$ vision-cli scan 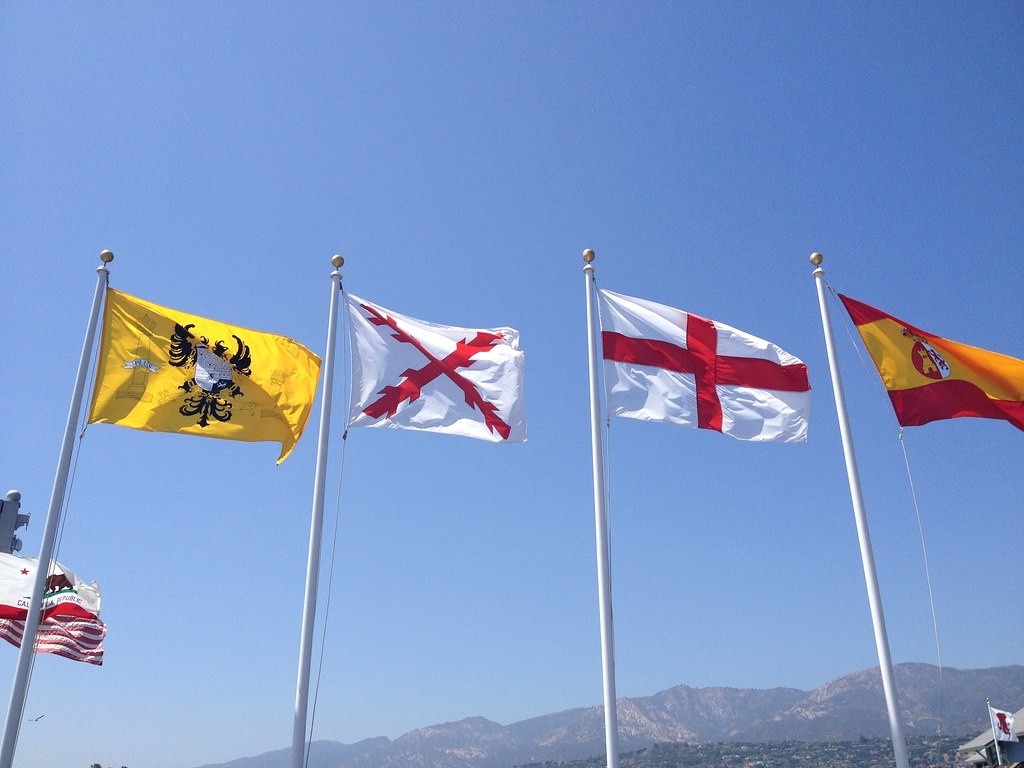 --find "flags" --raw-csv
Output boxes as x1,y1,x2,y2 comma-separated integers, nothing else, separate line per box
338,289,531,443
595,285,813,444
834,287,1024,434
0,549,106,666
88,288,323,467
990,707,1019,743
